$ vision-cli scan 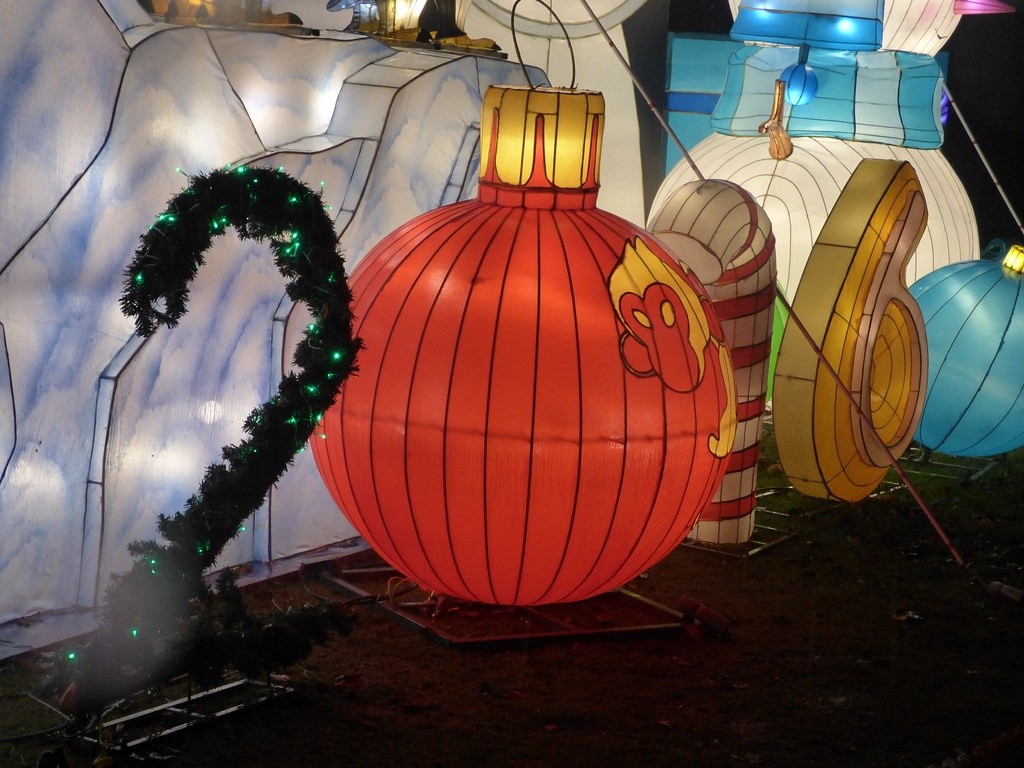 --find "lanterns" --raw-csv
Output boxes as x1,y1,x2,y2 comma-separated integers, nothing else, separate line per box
303,83,737,608
907,243,1024,456
